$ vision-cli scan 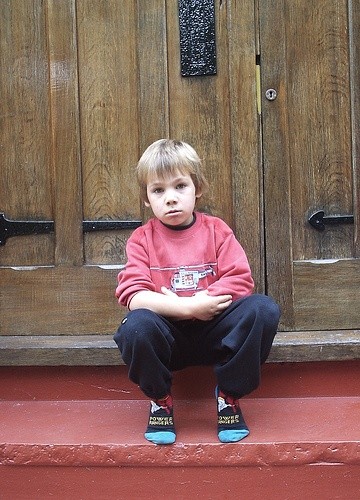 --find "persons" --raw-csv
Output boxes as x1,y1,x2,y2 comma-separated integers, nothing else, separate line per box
113,138,282,444
